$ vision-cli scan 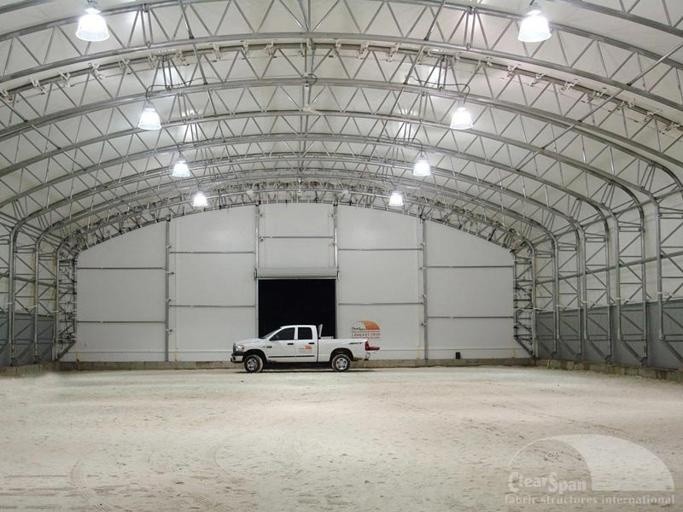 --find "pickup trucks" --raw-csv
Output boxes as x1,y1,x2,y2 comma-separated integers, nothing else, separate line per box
229,322,372,375
